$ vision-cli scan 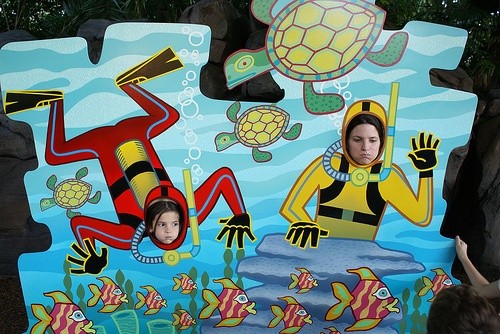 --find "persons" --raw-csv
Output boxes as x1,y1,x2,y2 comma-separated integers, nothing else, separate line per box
344,114,385,165
426,283,499,334
454,234,500,300
144,197,185,245
